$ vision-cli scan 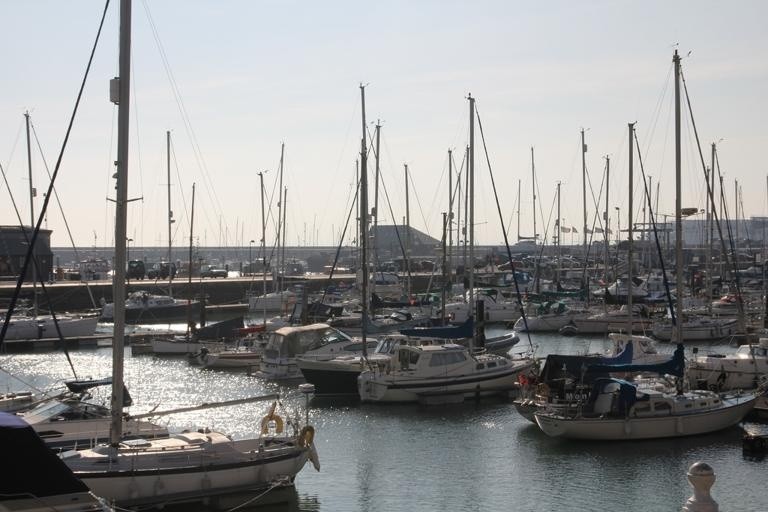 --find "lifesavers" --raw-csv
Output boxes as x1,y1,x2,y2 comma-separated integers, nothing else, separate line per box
262,415,283,435
298,426,314,446
